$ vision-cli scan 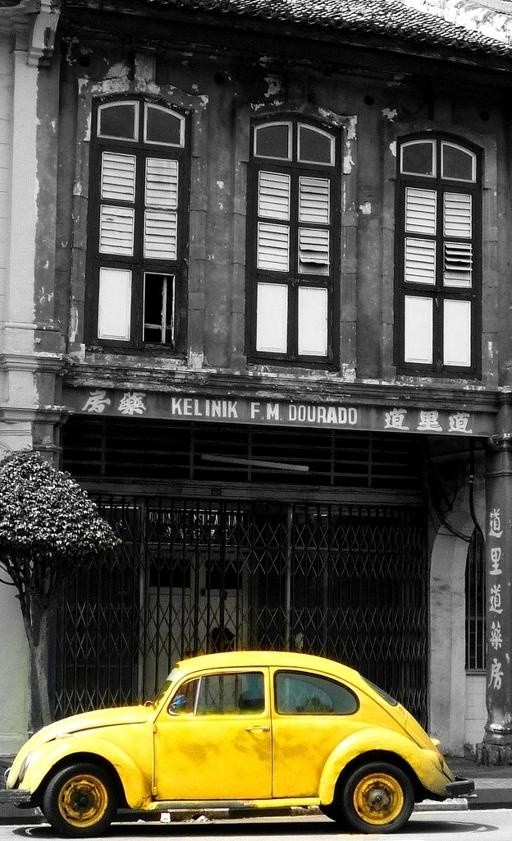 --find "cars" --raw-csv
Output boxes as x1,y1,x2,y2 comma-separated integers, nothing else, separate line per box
0,648,479,838
